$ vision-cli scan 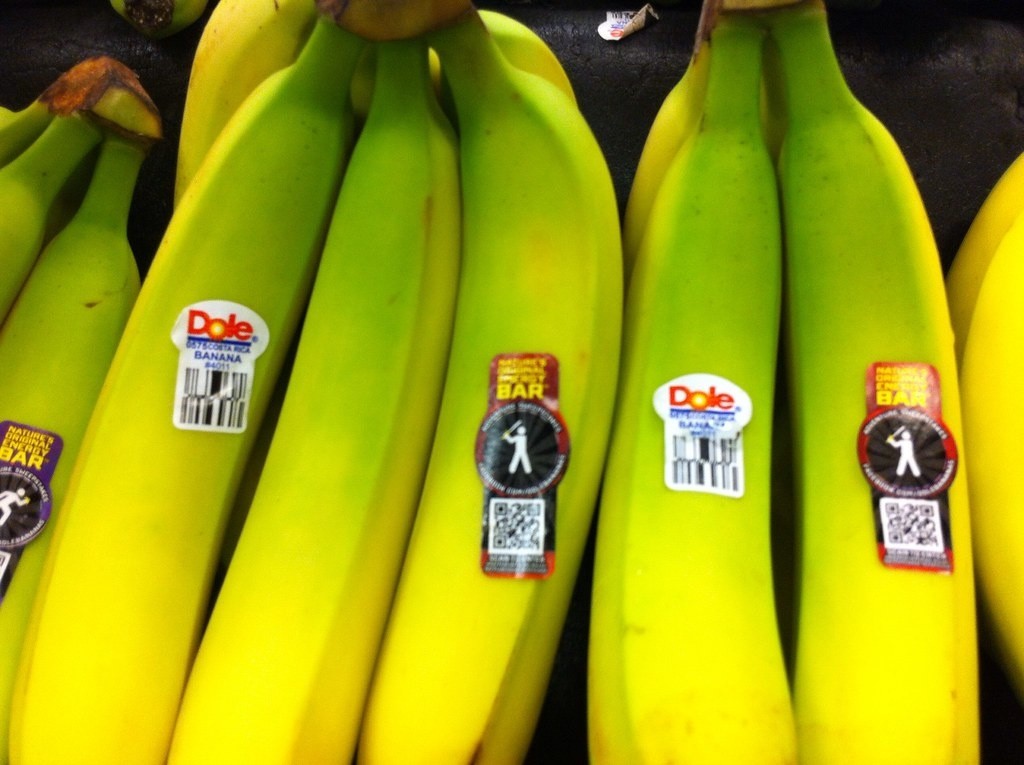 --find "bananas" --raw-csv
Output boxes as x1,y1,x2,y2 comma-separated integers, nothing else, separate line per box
0,0,1024,765
